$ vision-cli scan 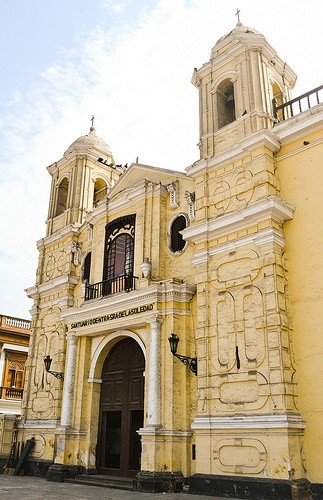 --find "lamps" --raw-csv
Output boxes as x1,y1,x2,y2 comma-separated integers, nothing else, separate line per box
168,333,198,376
43,355,64,380
80,279,91,298
140,257,153,279
